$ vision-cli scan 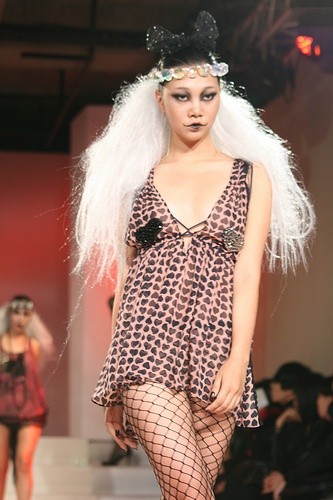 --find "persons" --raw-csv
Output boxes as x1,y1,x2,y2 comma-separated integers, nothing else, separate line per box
102,295,135,467
92,38,271,500
214,361,332,500
1,293,48,499
